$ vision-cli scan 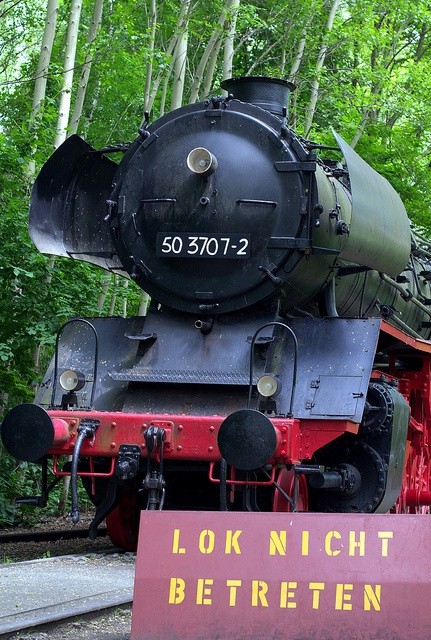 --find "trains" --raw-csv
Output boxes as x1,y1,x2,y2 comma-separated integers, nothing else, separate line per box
0,78,431,550
